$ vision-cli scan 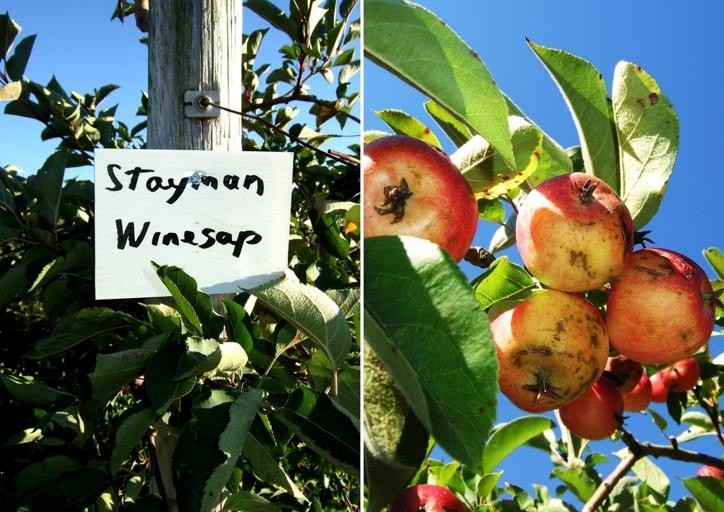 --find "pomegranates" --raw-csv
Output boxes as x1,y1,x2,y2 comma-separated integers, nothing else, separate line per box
362,136,723,512
